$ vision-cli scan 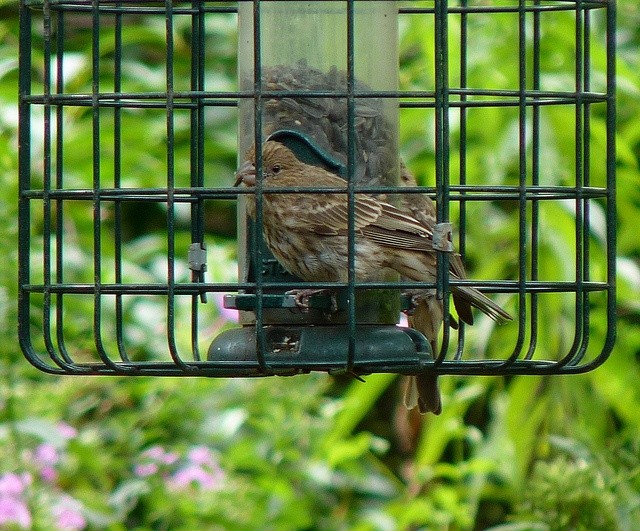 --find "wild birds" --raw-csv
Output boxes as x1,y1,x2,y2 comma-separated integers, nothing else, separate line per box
233,140,515,329
395,160,475,419
